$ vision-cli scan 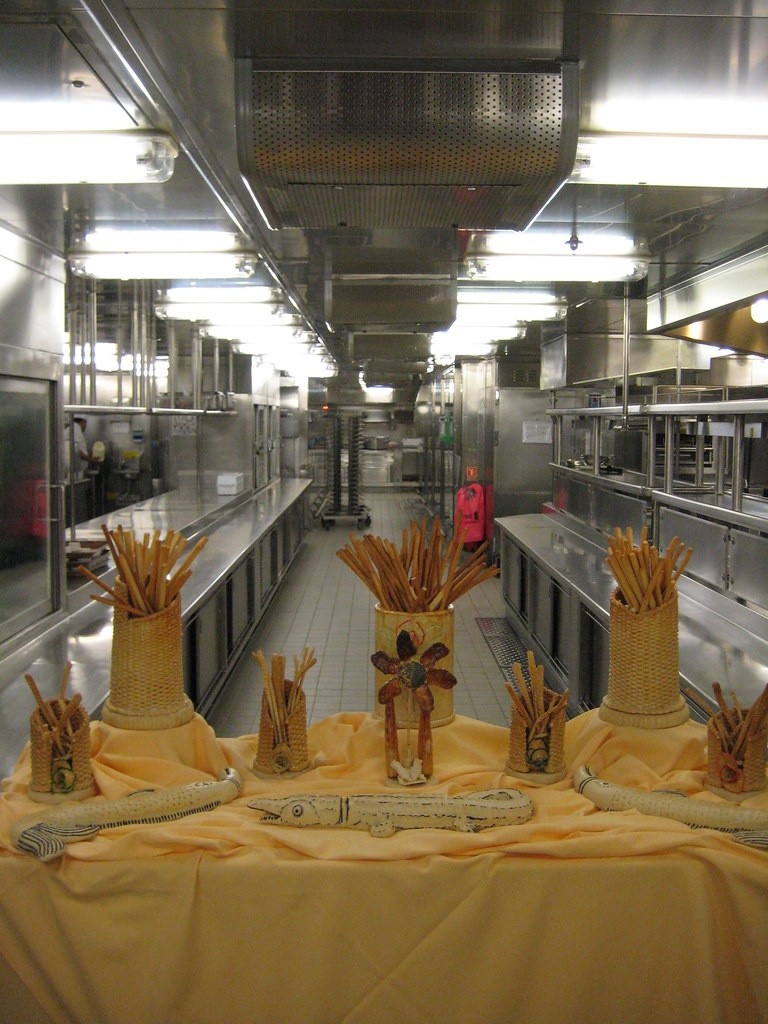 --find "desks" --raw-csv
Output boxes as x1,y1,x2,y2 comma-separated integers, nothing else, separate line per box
0,722,768,1024
